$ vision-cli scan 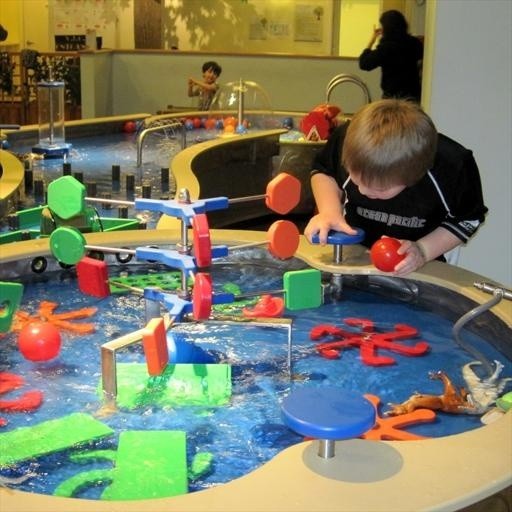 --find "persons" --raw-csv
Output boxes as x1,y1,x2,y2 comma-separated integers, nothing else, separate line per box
188,62,222,110
304,98,488,278
359,10,426,102
